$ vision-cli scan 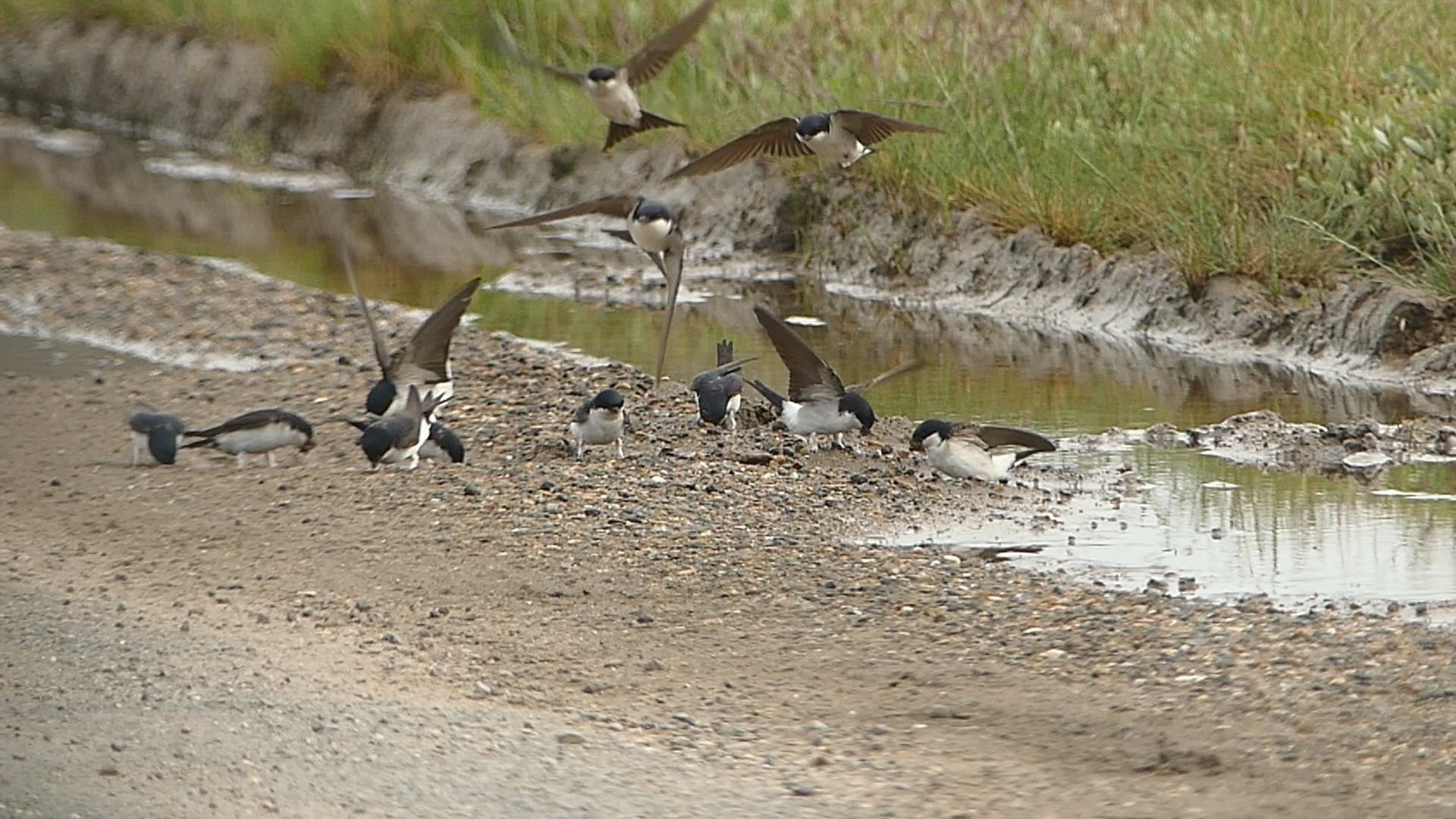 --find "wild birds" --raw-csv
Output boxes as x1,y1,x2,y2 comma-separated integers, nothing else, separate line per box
907,419,1055,486
128,412,184,468
689,341,759,431
484,190,689,390
363,389,455,472
752,304,927,436
315,248,480,466
660,109,946,183
180,409,315,470
487,0,717,153
568,391,625,459
